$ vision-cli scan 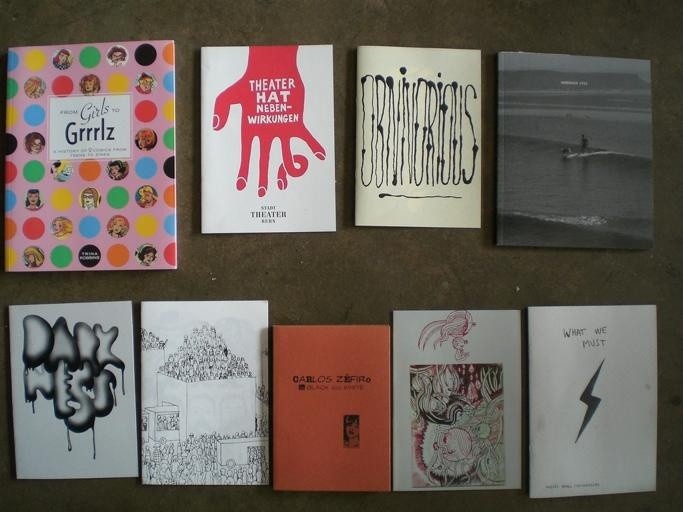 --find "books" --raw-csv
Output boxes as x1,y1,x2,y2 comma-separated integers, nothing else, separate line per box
391,308,526,489
7,299,140,480
269,324,394,495
528,302,659,499
1,38,177,273
494,50,653,251
353,40,480,229
194,43,338,234
140,298,271,488
